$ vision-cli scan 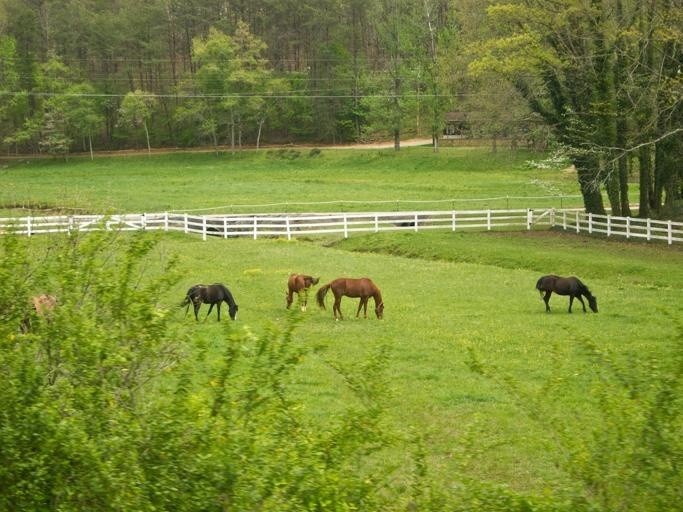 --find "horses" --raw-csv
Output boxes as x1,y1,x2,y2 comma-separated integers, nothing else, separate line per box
181,283,239,324
536,275,599,313
285,273,321,313
314,277,385,322
